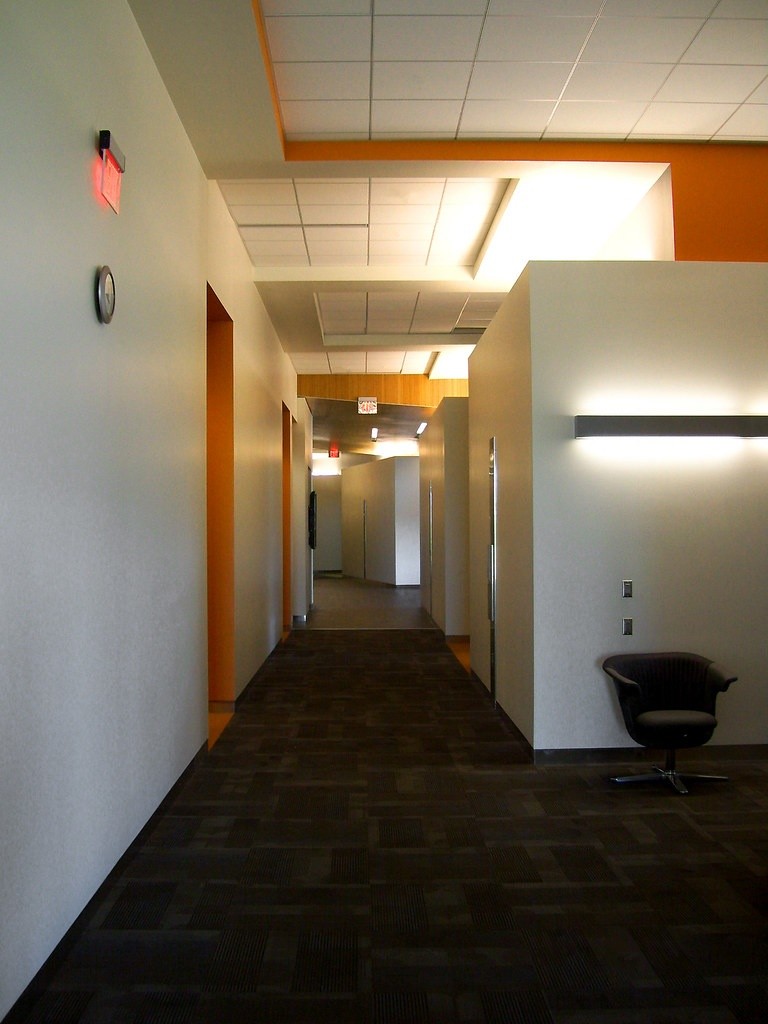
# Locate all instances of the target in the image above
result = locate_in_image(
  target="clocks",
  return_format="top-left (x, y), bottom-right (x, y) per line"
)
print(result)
top-left (96, 264), bottom-right (116, 324)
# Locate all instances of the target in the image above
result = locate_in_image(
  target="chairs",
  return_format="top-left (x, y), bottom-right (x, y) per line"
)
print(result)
top-left (603, 652), bottom-right (739, 794)
